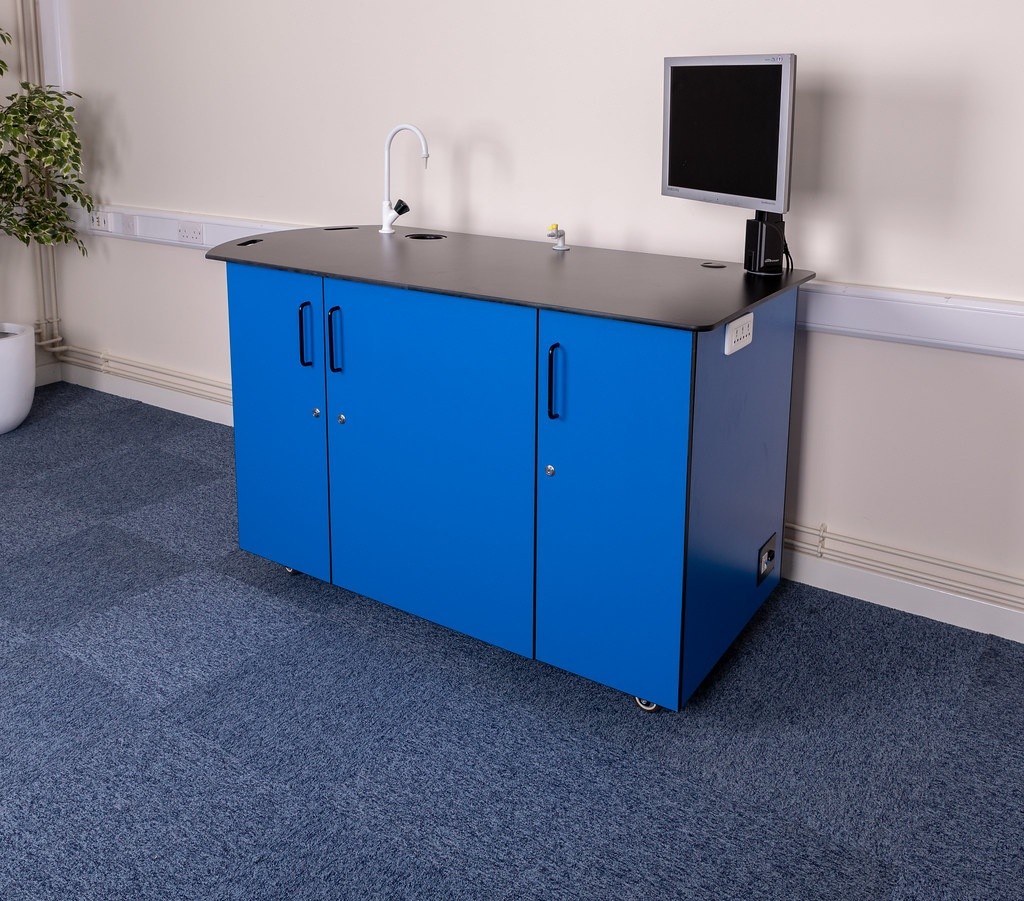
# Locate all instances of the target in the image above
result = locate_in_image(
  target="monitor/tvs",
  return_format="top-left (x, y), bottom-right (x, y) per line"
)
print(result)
top-left (661, 53), bottom-right (797, 213)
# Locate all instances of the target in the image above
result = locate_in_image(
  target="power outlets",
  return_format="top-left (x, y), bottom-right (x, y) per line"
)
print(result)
top-left (178, 221), bottom-right (203, 243)
top-left (90, 211), bottom-right (113, 231)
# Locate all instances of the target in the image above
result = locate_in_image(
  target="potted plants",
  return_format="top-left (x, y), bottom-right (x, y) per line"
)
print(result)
top-left (0, 30), bottom-right (98, 434)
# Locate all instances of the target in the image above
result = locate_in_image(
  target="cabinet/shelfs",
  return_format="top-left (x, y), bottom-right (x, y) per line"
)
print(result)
top-left (205, 223), bottom-right (816, 714)
